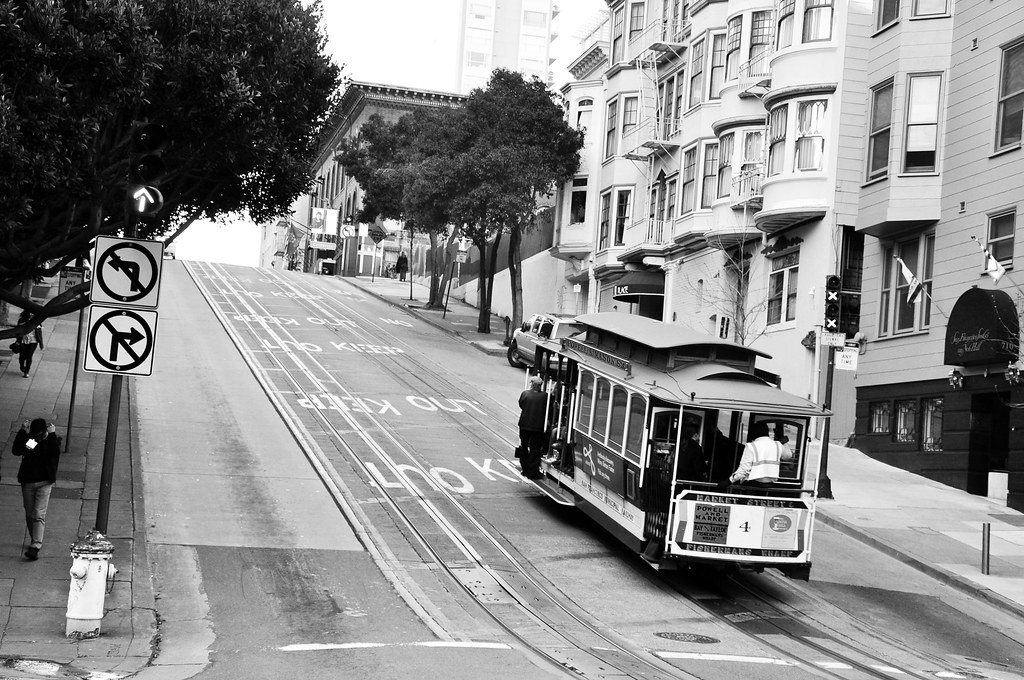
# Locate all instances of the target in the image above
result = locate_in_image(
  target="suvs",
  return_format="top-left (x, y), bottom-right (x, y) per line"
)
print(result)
top-left (504, 312), bottom-right (581, 375)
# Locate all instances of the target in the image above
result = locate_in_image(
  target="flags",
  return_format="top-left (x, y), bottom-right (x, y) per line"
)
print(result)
top-left (975, 238), bottom-right (1006, 286)
top-left (896, 257), bottom-right (923, 307)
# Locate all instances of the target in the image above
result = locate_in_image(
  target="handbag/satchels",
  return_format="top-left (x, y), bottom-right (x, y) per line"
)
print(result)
top-left (9, 342), bottom-right (20, 354)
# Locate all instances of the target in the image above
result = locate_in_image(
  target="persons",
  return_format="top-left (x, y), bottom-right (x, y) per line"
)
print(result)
top-left (12, 419), bottom-right (61, 559)
top-left (717, 421), bottom-right (793, 505)
top-left (667, 417), bottom-right (705, 502)
top-left (16, 309), bottom-right (44, 378)
top-left (396, 252), bottom-right (409, 282)
top-left (518, 376), bottom-right (569, 479)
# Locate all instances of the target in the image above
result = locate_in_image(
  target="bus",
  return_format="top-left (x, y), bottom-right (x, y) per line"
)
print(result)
top-left (513, 310), bottom-right (835, 582)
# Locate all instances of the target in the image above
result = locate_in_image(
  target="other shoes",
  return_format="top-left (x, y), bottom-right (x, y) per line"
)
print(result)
top-left (526, 472), bottom-right (543, 479)
top-left (20, 366), bottom-right (25, 372)
top-left (24, 373), bottom-right (29, 378)
top-left (24, 546), bottom-right (39, 561)
top-left (520, 470), bottom-right (527, 476)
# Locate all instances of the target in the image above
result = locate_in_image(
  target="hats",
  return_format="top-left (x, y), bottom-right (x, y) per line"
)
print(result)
top-left (530, 376), bottom-right (543, 385)
top-left (28, 418), bottom-right (47, 441)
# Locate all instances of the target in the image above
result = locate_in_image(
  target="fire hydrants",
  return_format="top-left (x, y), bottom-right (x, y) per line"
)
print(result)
top-left (64, 527), bottom-right (118, 641)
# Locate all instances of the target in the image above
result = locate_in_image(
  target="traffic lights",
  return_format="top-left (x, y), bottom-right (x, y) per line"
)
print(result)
top-left (825, 271), bottom-right (843, 335)
top-left (127, 117), bottom-right (171, 219)
